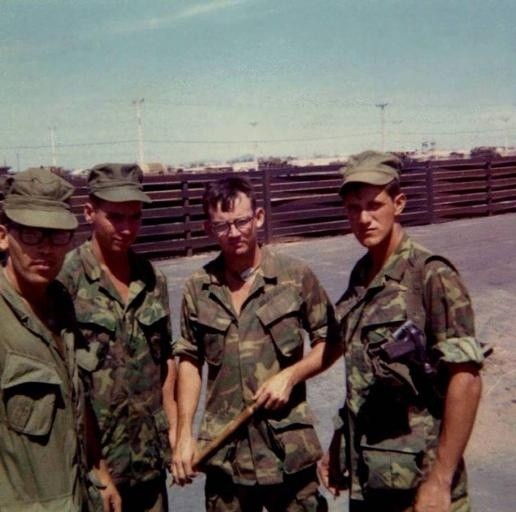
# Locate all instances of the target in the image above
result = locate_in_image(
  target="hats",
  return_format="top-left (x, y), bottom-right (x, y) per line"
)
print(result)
top-left (2, 166), bottom-right (80, 232)
top-left (86, 162), bottom-right (154, 206)
top-left (338, 150), bottom-right (405, 196)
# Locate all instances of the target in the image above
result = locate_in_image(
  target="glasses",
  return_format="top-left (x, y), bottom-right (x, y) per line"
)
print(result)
top-left (209, 213), bottom-right (255, 238)
top-left (16, 226), bottom-right (75, 248)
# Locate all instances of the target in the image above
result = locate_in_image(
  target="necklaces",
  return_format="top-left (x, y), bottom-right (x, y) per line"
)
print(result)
top-left (228, 261), bottom-right (258, 281)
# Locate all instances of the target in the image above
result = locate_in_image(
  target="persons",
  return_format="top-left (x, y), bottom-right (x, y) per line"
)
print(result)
top-left (53, 161), bottom-right (177, 512)
top-left (319, 150), bottom-right (484, 512)
top-left (168, 175), bottom-right (344, 512)
top-left (0, 167), bottom-right (122, 512)
